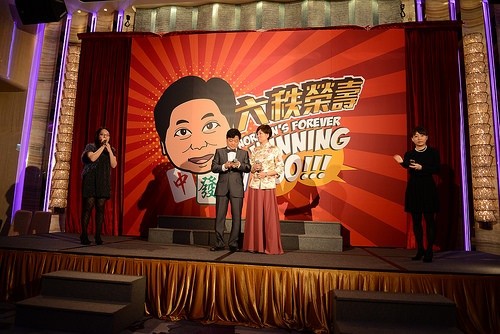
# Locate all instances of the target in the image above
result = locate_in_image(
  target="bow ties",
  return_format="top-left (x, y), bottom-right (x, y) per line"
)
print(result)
top-left (229, 149), bottom-right (236, 152)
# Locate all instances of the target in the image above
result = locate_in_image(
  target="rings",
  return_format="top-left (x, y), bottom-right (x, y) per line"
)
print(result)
top-left (259, 177), bottom-right (260, 179)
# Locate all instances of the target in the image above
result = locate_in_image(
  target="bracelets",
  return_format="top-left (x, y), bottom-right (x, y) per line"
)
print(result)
top-left (266, 172), bottom-right (268, 176)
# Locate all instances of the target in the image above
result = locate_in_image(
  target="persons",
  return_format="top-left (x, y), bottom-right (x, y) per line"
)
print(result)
top-left (242, 124), bottom-right (284, 255)
top-left (81, 128), bottom-right (117, 245)
top-left (394, 127), bottom-right (440, 263)
top-left (210, 129), bottom-right (252, 252)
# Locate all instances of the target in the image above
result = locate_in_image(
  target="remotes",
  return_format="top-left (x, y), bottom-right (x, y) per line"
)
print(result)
top-left (410, 159), bottom-right (415, 166)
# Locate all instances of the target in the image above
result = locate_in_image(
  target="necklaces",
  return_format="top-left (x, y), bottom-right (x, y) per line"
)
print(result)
top-left (415, 146), bottom-right (427, 152)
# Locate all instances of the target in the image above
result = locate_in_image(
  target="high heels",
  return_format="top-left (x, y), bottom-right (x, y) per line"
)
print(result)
top-left (412, 248), bottom-right (425, 260)
top-left (423, 248), bottom-right (433, 263)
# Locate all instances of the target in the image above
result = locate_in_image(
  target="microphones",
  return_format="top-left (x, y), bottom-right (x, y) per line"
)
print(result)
top-left (104, 140), bottom-right (106, 151)
top-left (256, 160), bottom-right (260, 173)
top-left (232, 159), bottom-right (237, 163)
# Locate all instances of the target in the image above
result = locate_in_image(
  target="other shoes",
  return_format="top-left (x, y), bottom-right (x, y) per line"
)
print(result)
top-left (81, 234), bottom-right (90, 245)
top-left (210, 245), bottom-right (225, 251)
top-left (95, 234), bottom-right (103, 245)
top-left (230, 247), bottom-right (239, 252)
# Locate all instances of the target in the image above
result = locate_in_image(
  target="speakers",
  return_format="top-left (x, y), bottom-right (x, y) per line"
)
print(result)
top-left (15, 0), bottom-right (68, 26)
